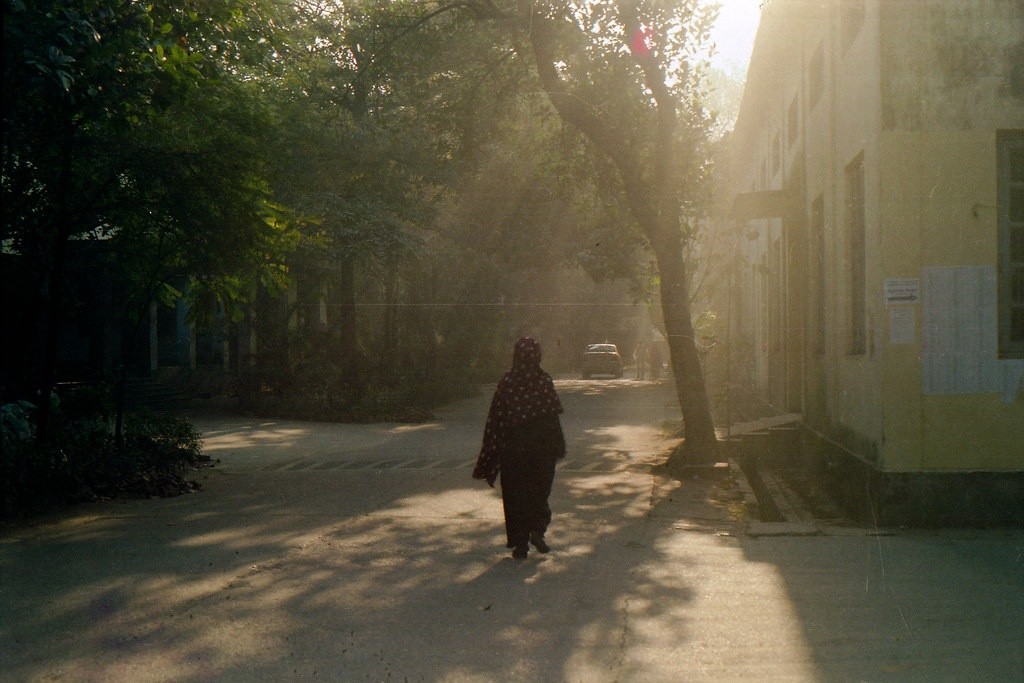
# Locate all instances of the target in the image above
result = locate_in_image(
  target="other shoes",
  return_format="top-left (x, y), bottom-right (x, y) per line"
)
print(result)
top-left (513, 547), bottom-right (527, 558)
top-left (530, 532), bottom-right (549, 553)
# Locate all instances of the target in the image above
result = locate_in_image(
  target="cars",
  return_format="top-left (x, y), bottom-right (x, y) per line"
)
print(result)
top-left (582, 343), bottom-right (624, 379)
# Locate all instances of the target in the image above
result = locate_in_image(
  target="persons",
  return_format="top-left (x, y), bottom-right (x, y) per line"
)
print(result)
top-left (472, 335), bottom-right (567, 558)
top-left (633, 341), bottom-right (663, 380)
